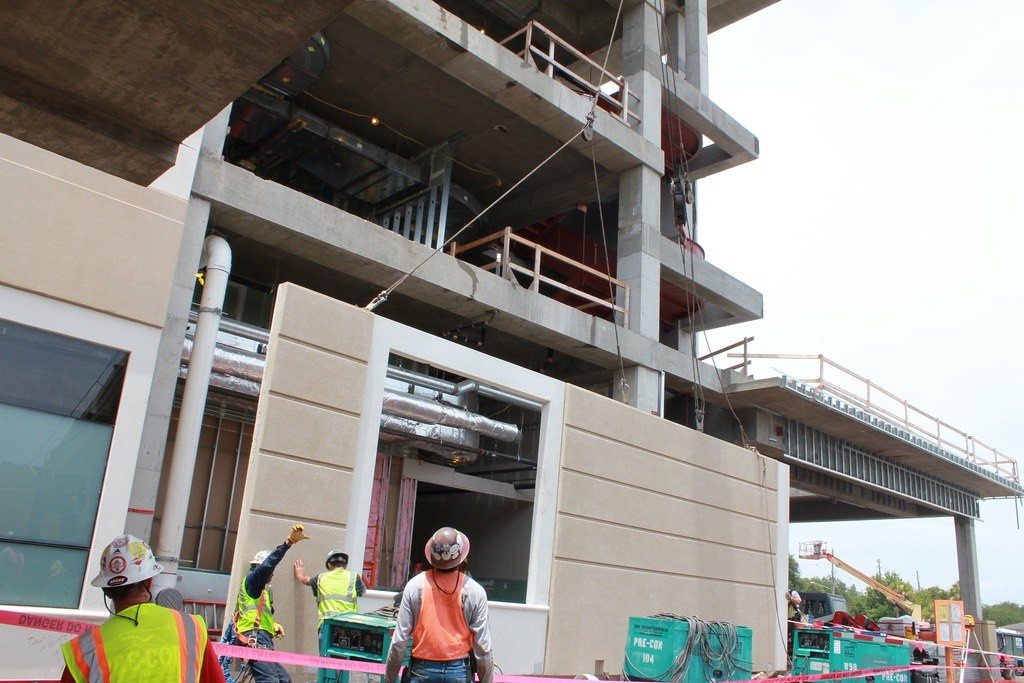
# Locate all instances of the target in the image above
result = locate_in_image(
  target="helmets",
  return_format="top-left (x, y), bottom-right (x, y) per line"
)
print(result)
top-left (250, 550), bottom-right (272, 564)
top-left (91, 534), bottom-right (164, 587)
top-left (326, 549), bottom-right (349, 571)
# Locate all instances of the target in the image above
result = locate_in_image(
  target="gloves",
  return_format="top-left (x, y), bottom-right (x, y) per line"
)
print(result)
top-left (274, 622), bottom-right (285, 639)
top-left (288, 524), bottom-right (311, 544)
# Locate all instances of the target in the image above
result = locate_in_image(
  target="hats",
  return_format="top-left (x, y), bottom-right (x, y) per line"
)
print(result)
top-left (425, 527), bottom-right (470, 569)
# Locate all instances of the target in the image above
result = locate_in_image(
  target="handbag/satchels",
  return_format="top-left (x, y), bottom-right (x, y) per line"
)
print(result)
top-left (793, 611), bottom-right (809, 624)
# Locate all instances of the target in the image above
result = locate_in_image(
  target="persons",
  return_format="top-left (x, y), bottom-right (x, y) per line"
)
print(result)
top-left (785, 589), bottom-right (801, 647)
top-left (60, 534), bottom-right (227, 683)
top-left (294, 547), bottom-right (366, 658)
top-left (232, 524), bottom-right (310, 683)
top-left (383, 527), bottom-right (495, 683)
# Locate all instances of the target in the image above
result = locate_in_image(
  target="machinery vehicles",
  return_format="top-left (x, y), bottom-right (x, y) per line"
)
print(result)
top-left (798, 540), bottom-right (932, 635)
top-left (788, 591), bottom-right (882, 658)
top-left (996, 627), bottom-right (1024, 680)
